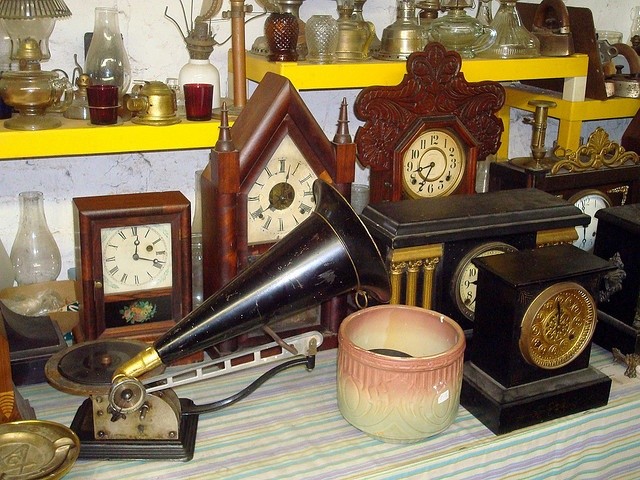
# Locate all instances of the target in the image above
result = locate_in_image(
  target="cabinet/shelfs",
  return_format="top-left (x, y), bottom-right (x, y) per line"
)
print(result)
top-left (2, 4), bottom-right (639, 157)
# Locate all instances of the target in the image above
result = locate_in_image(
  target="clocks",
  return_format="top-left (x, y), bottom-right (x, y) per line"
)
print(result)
top-left (199, 72), bottom-right (356, 368)
top-left (459, 244), bottom-right (617, 435)
top-left (353, 42), bottom-right (505, 203)
top-left (489, 101), bottom-right (639, 255)
top-left (359, 188), bottom-right (591, 344)
top-left (72, 190), bottom-right (205, 364)
